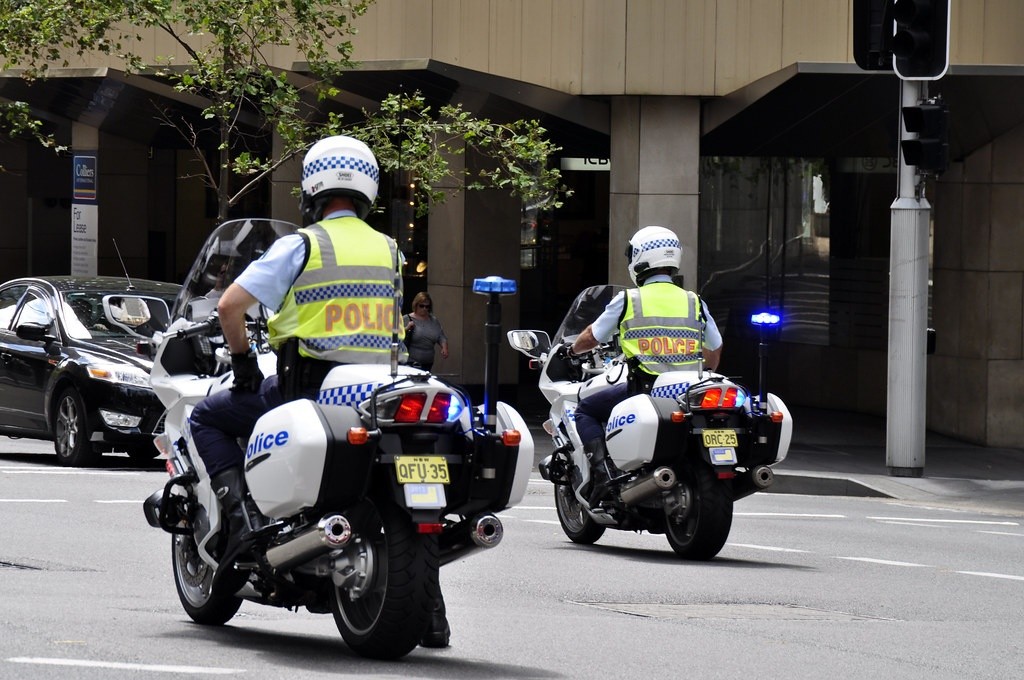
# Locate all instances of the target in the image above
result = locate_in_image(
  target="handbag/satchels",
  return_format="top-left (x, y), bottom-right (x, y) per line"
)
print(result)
top-left (404, 313), bottom-right (414, 349)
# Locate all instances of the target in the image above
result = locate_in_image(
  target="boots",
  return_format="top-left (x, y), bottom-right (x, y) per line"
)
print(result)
top-left (211, 467), bottom-right (273, 597)
top-left (583, 435), bottom-right (620, 509)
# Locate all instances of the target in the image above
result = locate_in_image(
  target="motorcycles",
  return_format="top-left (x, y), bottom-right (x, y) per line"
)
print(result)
top-left (102, 217), bottom-right (535, 661)
top-left (507, 284), bottom-right (794, 561)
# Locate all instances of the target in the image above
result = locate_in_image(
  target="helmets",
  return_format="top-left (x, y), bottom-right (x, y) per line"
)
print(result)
top-left (299, 134), bottom-right (380, 226)
top-left (628, 225), bottom-right (683, 288)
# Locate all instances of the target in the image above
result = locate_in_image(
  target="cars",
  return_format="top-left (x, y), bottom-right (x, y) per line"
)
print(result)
top-left (0, 276), bottom-right (185, 468)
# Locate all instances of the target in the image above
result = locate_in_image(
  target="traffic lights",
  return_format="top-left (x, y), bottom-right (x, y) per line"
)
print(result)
top-left (900, 139), bottom-right (942, 166)
top-left (900, 106), bottom-right (942, 133)
top-left (889, 0), bottom-right (950, 81)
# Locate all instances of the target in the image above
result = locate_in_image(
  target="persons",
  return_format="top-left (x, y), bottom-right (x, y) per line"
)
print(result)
top-left (191, 136), bottom-right (399, 596)
top-left (554, 225), bottom-right (726, 513)
top-left (401, 292), bottom-right (450, 372)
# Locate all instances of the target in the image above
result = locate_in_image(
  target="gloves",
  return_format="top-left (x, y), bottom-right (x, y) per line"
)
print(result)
top-left (556, 342), bottom-right (578, 361)
top-left (229, 347), bottom-right (264, 395)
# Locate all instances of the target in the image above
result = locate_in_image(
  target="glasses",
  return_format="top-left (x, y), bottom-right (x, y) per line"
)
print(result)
top-left (418, 303), bottom-right (431, 309)
